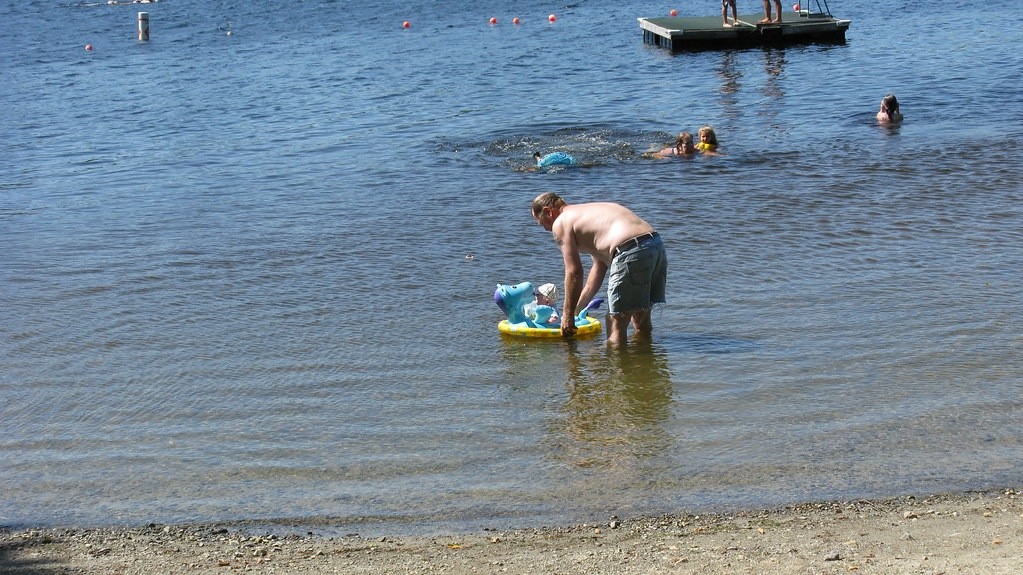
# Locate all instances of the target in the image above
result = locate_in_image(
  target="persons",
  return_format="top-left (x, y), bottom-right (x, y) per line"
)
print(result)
top-left (533, 283), bottom-right (561, 324)
top-left (531, 192), bottom-right (667, 348)
top-left (877, 94), bottom-right (904, 126)
top-left (721, 0), bottom-right (742, 28)
top-left (758, 0), bottom-right (783, 24)
top-left (652, 125), bottom-right (721, 159)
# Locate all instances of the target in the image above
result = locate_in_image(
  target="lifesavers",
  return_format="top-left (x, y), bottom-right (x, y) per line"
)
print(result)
top-left (537, 150), bottom-right (575, 167)
top-left (492, 281), bottom-right (606, 338)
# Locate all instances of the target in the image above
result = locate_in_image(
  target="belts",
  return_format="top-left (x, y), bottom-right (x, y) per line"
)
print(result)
top-left (613, 232), bottom-right (658, 257)
